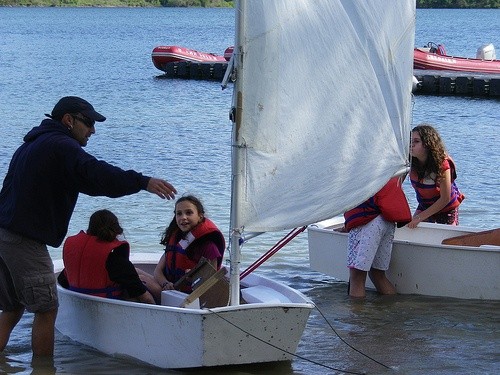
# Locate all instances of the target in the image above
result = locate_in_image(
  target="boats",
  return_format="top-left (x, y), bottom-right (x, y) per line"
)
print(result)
top-left (414, 41), bottom-right (500, 99)
top-left (151, 45), bottom-right (235, 79)
top-left (308, 211), bottom-right (500, 301)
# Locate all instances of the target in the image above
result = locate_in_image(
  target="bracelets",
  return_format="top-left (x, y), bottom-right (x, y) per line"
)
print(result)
top-left (414, 215), bottom-right (421, 221)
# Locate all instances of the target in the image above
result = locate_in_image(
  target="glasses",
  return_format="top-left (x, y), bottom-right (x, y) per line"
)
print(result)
top-left (71, 115), bottom-right (95, 128)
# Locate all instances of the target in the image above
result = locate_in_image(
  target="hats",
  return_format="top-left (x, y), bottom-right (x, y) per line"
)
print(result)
top-left (51, 96), bottom-right (106, 122)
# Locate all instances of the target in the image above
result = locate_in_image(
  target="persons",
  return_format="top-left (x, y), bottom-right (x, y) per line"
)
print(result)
top-left (135, 196), bottom-right (226, 303)
top-left (333, 196), bottom-right (396, 295)
top-left (406, 125), bottom-right (465, 228)
top-left (63, 209), bottom-right (153, 302)
top-left (0, 96), bottom-right (177, 375)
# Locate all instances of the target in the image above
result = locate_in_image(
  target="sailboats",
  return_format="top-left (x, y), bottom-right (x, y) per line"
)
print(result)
top-left (52, 0), bottom-right (422, 370)
